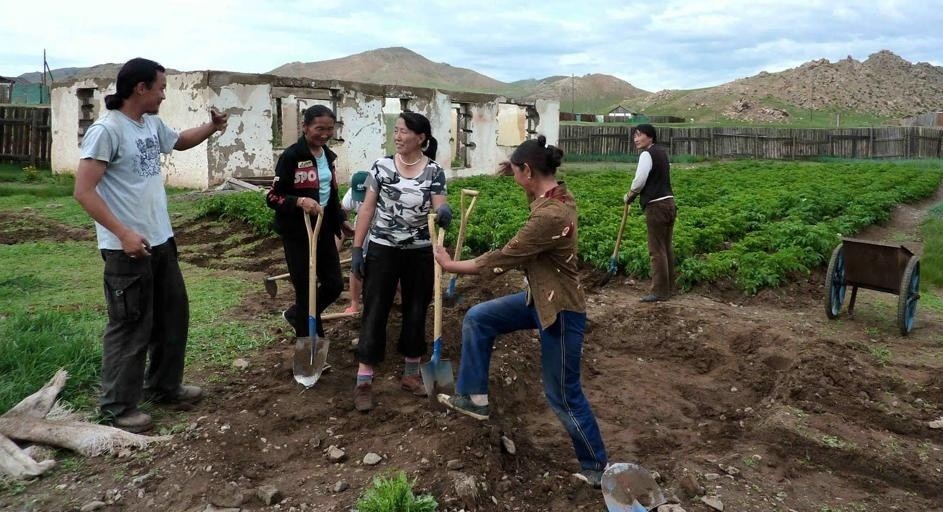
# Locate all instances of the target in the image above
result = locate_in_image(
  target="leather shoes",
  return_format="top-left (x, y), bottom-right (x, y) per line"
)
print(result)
top-left (168, 385), bottom-right (204, 403)
top-left (113, 409), bottom-right (154, 433)
top-left (639, 294), bottom-right (656, 303)
top-left (353, 383), bottom-right (374, 411)
top-left (401, 374), bottom-right (427, 396)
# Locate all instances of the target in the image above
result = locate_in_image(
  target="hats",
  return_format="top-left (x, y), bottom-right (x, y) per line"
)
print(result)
top-left (351, 171), bottom-right (369, 201)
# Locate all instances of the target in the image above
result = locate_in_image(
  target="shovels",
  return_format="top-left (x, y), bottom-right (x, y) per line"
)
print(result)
top-left (420, 214), bottom-right (455, 413)
top-left (600, 463), bottom-right (668, 512)
top-left (447, 189), bottom-right (480, 297)
top-left (600, 203), bottom-right (631, 287)
top-left (293, 205), bottom-right (330, 389)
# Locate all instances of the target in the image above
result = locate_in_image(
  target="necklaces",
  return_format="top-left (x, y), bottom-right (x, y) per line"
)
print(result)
top-left (398, 153), bottom-right (423, 167)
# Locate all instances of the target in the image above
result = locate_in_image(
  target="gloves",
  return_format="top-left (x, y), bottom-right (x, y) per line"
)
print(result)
top-left (623, 192), bottom-right (632, 204)
top-left (351, 247), bottom-right (367, 282)
top-left (434, 204), bottom-right (453, 230)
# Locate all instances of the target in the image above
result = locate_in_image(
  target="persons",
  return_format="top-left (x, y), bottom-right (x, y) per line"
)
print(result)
top-left (623, 123), bottom-right (678, 300)
top-left (73, 57), bottom-right (229, 432)
top-left (334, 170), bottom-right (402, 315)
top-left (432, 133), bottom-right (608, 487)
top-left (349, 107), bottom-right (453, 413)
top-left (265, 105), bottom-right (345, 373)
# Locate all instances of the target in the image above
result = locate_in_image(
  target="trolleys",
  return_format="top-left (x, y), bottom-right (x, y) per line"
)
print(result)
top-left (824, 236), bottom-right (922, 336)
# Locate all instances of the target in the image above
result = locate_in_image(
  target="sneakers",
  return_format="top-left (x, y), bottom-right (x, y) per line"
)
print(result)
top-left (282, 304), bottom-right (298, 333)
top-left (571, 462), bottom-right (609, 487)
top-left (322, 362), bottom-right (332, 373)
top-left (345, 306), bottom-right (358, 313)
top-left (437, 393), bottom-right (490, 420)
